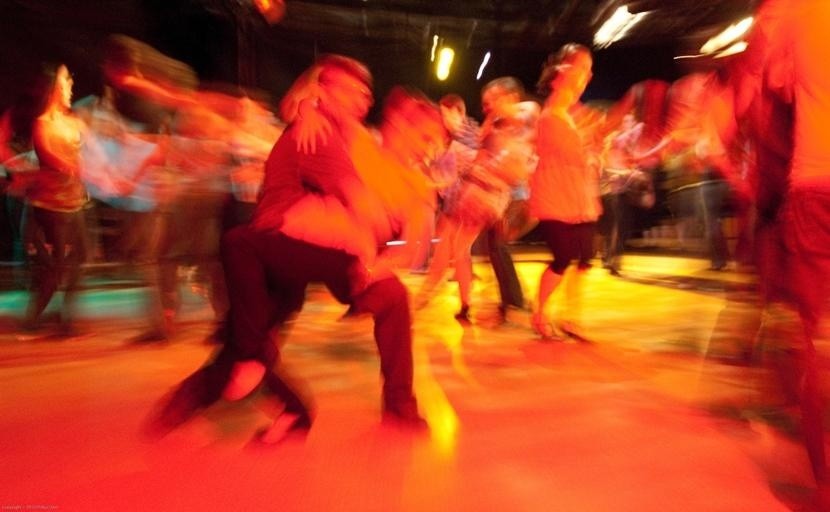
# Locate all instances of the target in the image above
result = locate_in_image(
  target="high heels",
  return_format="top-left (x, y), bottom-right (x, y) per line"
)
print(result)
top-left (455, 304), bottom-right (470, 319)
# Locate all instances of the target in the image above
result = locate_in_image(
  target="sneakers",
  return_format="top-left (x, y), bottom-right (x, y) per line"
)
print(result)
top-left (532, 313), bottom-right (592, 343)
